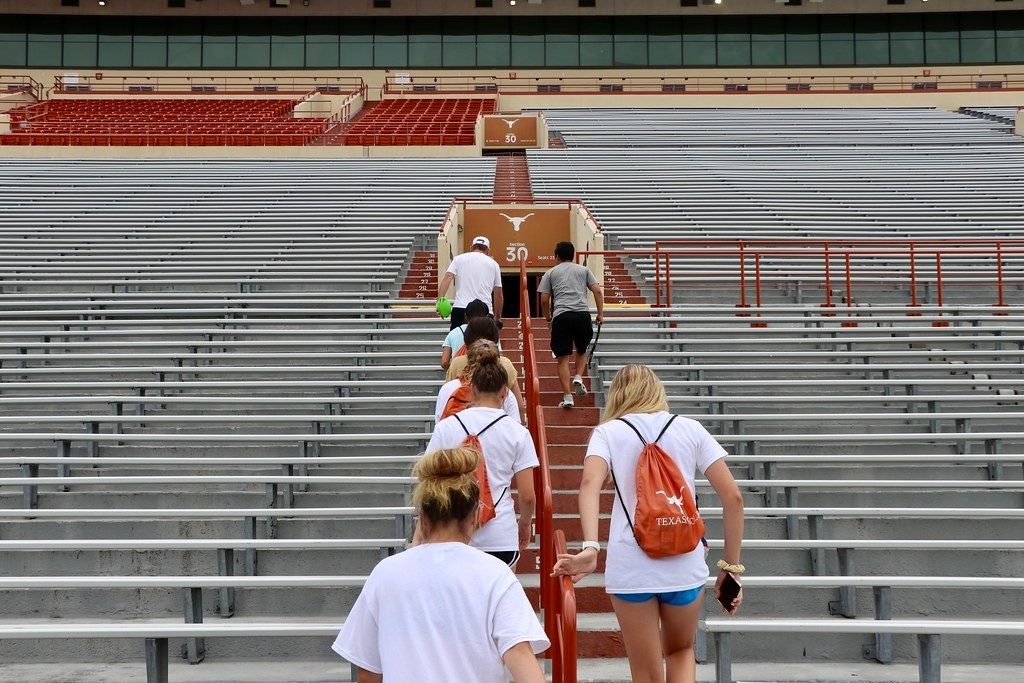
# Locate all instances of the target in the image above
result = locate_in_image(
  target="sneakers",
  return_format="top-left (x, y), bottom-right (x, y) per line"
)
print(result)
top-left (559, 395), bottom-right (574, 408)
top-left (572, 375), bottom-right (587, 399)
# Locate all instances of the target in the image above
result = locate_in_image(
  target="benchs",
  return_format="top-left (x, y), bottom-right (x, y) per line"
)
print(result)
top-left (0, 156), bottom-right (497, 682)
top-left (518, 103), bottom-right (1024, 683)
top-left (0, 99), bottom-right (498, 146)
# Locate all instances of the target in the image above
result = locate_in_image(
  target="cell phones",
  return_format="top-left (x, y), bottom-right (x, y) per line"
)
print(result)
top-left (717, 572), bottom-right (742, 613)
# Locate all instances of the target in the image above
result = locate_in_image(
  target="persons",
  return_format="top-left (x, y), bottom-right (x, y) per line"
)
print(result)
top-left (330, 446), bottom-right (552, 683)
top-left (537, 241), bottom-right (603, 408)
top-left (440, 297), bottom-right (503, 371)
top-left (411, 350), bottom-right (540, 574)
top-left (435, 235), bottom-right (503, 332)
top-left (436, 340), bottom-right (523, 424)
top-left (445, 317), bottom-right (526, 425)
top-left (550, 363), bottom-right (744, 683)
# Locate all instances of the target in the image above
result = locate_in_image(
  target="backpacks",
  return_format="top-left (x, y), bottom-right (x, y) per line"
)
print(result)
top-left (609, 413), bottom-right (706, 558)
top-left (452, 326), bottom-right (468, 359)
top-left (440, 374), bottom-right (477, 421)
top-left (453, 412), bottom-right (509, 531)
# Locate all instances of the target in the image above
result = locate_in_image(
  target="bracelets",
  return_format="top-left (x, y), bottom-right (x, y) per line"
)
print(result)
top-left (546, 318), bottom-right (553, 323)
top-left (717, 559), bottom-right (745, 573)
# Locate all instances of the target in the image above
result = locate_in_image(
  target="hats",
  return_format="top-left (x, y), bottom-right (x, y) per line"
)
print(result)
top-left (472, 236), bottom-right (489, 249)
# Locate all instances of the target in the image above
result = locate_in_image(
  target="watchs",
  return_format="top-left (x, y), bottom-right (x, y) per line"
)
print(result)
top-left (582, 541), bottom-right (600, 552)
